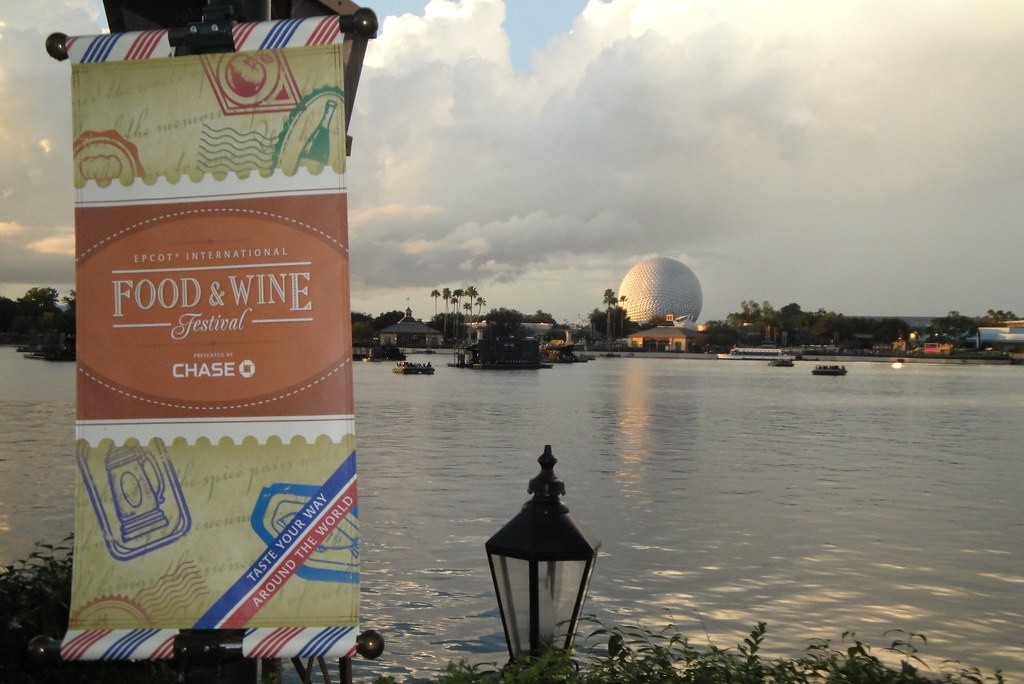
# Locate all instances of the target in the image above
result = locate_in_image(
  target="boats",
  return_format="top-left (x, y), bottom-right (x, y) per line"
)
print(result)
top-left (812, 364), bottom-right (848, 376)
top-left (767, 357), bottom-right (794, 367)
top-left (716, 347), bottom-right (793, 360)
top-left (392, 320), bottom-right (596, 374)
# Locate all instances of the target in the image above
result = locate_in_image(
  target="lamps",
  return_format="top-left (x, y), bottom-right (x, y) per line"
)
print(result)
top-left (486, 444), bottom-right (601, 683)
